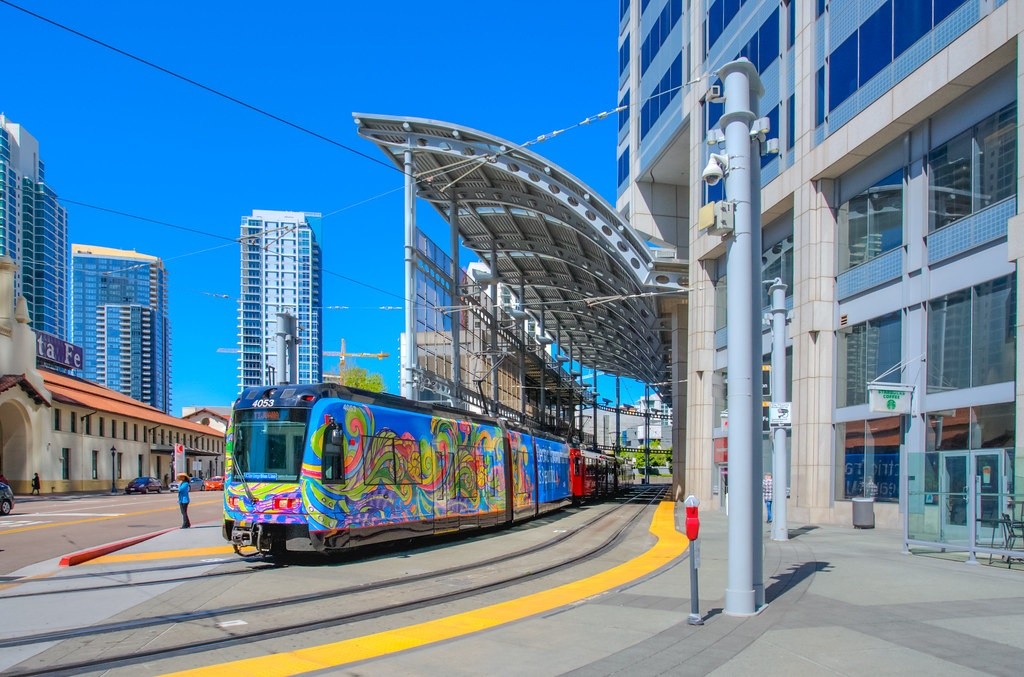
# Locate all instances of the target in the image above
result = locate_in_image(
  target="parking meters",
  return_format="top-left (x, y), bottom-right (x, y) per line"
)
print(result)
top-left (684, 493), bottom-right (704, 626)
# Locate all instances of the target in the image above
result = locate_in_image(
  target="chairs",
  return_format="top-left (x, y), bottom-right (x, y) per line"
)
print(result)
top-left (1004, 514), bottom-right (1024, 569)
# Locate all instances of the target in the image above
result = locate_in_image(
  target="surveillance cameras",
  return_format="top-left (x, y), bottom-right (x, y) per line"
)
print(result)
top-left (707, 86), bottom-right (720, 102)
top-left (702, 164), bottom-right (723, 186)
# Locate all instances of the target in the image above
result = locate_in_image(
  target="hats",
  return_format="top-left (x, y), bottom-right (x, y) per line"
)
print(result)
top-left (177, 472), bottom-right (190, 481)
top-left (34, 473), bottom-right (38, 475)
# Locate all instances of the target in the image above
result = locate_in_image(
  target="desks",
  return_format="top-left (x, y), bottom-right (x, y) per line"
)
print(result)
top-left (975, 518), bottom-right (1024, 563)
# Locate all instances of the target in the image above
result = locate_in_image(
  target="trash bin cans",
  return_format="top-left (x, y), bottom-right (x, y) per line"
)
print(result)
top-left (851, 497), bottom-right (875, 529)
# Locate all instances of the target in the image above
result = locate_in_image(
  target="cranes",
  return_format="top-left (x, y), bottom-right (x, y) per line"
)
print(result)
top-left (322, 338), bottom-right (390, 385)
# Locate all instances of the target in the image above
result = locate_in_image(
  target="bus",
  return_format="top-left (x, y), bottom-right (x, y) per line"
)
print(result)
top-left (221, 381), bottom-right (572, 561)
top-left (570, 449), bottom-right (633, 504)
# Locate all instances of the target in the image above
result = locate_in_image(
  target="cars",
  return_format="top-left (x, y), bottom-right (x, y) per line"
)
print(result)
top-left (168, 476), bottom-right (205, 493)
top-left (0, 482), bottom-right (16, 516)
top-left (126, 476), bottom-right (162, 493)
top-left (204, 476), bottom-right (224, 491)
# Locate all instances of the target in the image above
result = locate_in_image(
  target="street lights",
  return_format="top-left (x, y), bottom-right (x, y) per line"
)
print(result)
top-left (110, 445), bottom-right (118, 493)
top-left (215, 457), bottom-right (218, 476)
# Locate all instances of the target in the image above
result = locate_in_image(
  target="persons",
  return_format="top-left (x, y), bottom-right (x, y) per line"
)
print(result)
top-left (164, 474), bottom-right (169, 487)
top-left (762, 473), bottom-right (773, 523)
top-left (30, 473), bottom-right (40, 495)
top-left (177, 472), bottom-right (191, 528)
top-left (0, 475), bottom-right (6, 482)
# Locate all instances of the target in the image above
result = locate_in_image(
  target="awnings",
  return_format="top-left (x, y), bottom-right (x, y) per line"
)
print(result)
top-left (150, 444), bottom-right (222, 476)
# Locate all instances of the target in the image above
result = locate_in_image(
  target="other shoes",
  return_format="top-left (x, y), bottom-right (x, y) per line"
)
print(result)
top-left (180, 525), bottom-right (191, 529)
top-left (36, 493), bottom-right (40, 496)
top-left (30, 493), bottom-right (33, 495)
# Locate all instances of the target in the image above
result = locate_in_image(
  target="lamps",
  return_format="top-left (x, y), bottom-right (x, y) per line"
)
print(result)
top-left (498, 308), bottom-right (531, 330)
top-left (563, 369), bottom-right (581, 383)
top-left (598, 398), bottom-right (613, 407)
top-left (621, 404), bottom-right (634, 412)
top-left (574, 383), bottom-right (592, 393)
top-left (549, 354), bottom-right (570, 369)
top-left (651, 408), bottom-right (663, 414)
top-left (526, 334), bottom-right (553, 353)
top-left (586, 392), bottom-right (601, 400)
top-left (458, 269), bottom-right (497, 299)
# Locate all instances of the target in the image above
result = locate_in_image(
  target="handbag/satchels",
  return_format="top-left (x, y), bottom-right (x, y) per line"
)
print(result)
top-left (32, 483), bottom-right (35, 487)
